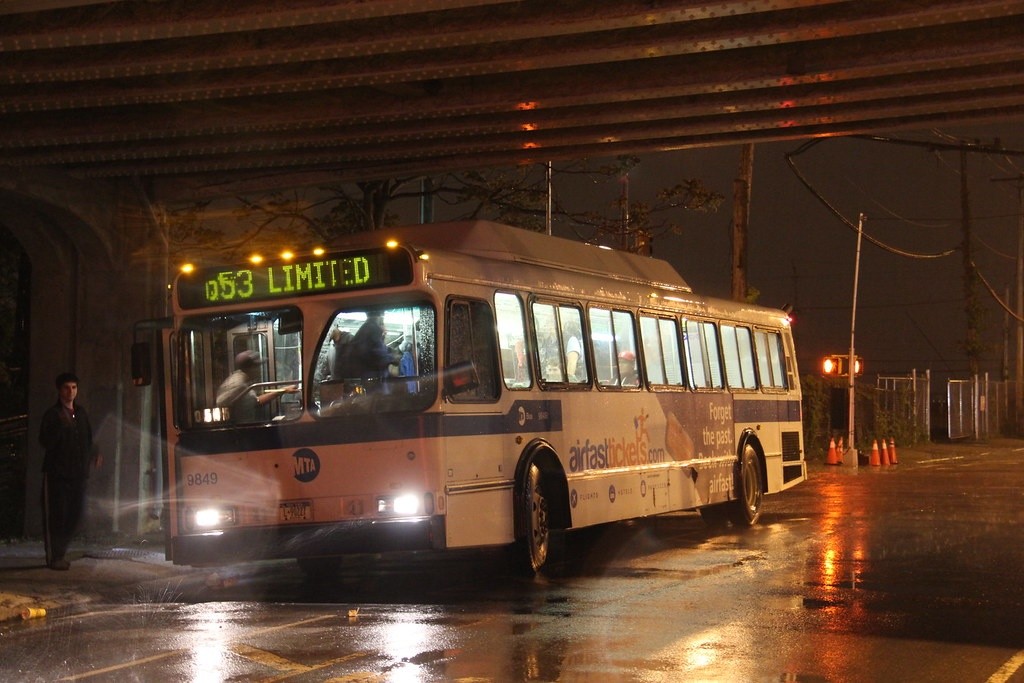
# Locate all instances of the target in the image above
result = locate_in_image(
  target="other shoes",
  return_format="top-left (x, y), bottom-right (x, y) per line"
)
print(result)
top-left (48, 556), bottom-right (70, 570)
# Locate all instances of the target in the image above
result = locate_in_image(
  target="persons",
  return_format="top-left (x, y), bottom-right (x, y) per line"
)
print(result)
top-left (40, 371), bottom-right (102, 571)
top-left (329, 308), bottom-right (420, 399)
top-left (216, 351), bottom-right (302, 424)
top-left (512, 314), bottom-right (588, 383)
top-left (608, 350), bottom-right (638, 385)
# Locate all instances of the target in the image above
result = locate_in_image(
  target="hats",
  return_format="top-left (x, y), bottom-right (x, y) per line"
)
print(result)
top-left (55, 372), bottom-right (81, 386)
top-left (235, 349), bottom-right (267, 368)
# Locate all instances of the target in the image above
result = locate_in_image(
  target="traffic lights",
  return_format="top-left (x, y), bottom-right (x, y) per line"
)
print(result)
top-left (820, 354), bottom-right (863, 378)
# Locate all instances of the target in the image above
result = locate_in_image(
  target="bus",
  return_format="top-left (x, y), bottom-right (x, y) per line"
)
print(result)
top-left (131, 220), bottom-right (808, 577)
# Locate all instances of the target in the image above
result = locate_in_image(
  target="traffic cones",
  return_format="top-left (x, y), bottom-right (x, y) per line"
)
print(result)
top-left (888, 437), bottom-right (898, 464)
top-left (870, 439), bottom-right (881, 465)
top-left (824, 437), bottom-right (844, 465)
top-left (881, 438), bottom-right (890, 464)
